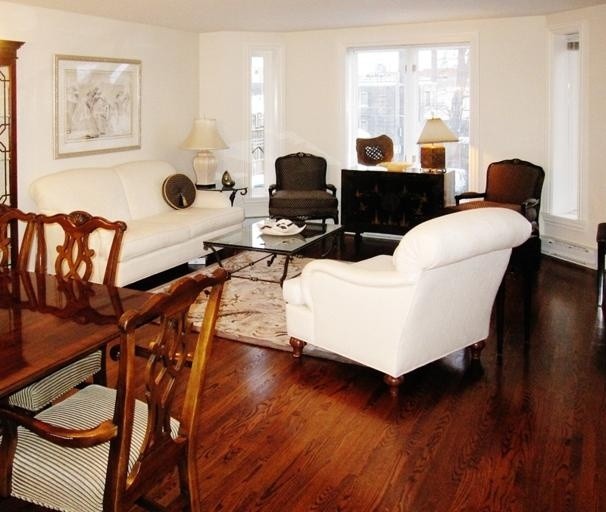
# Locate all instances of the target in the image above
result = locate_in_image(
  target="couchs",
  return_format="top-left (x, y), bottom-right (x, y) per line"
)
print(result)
top-left (281, 208), bottom-right (534, 399)
top-left (26, 158), bottom-right (246, 291)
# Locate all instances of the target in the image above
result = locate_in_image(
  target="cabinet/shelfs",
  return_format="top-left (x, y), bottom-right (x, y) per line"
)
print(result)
top-left (341, 164), bottom-right (455, 243)
top-left (2, 40), bottom-right (25, 271)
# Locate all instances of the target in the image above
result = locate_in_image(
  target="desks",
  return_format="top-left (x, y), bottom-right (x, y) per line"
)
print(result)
top-left (206, 183), bottom-right (248, 207)
top-left (1, 264), bottom-right (162, 404)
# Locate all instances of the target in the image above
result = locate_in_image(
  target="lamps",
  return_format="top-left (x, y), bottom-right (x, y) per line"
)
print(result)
top-left (418, 115), bottom-right (461, 173)
top-left (180, 115), bottom-right (230, 189)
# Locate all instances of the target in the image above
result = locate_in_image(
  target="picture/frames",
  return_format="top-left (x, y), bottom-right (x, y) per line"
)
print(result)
top-left (52, 52), bottom-right (144, 161)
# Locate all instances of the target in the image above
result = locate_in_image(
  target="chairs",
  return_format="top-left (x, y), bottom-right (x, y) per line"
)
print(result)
top-left (454, 156), bottom-right (545, 243)
top-left (266, 152), bottom-right (340, 225)
top-left (0, 204), bottom-right (39, 267)
top-left (5, 208), bottom-right (128, 416)
top-left (0, 268), bottom-right (227, 510)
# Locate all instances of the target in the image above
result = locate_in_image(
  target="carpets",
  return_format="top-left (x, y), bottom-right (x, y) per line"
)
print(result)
top-left (140, 250), bottom-right (363, 366)
